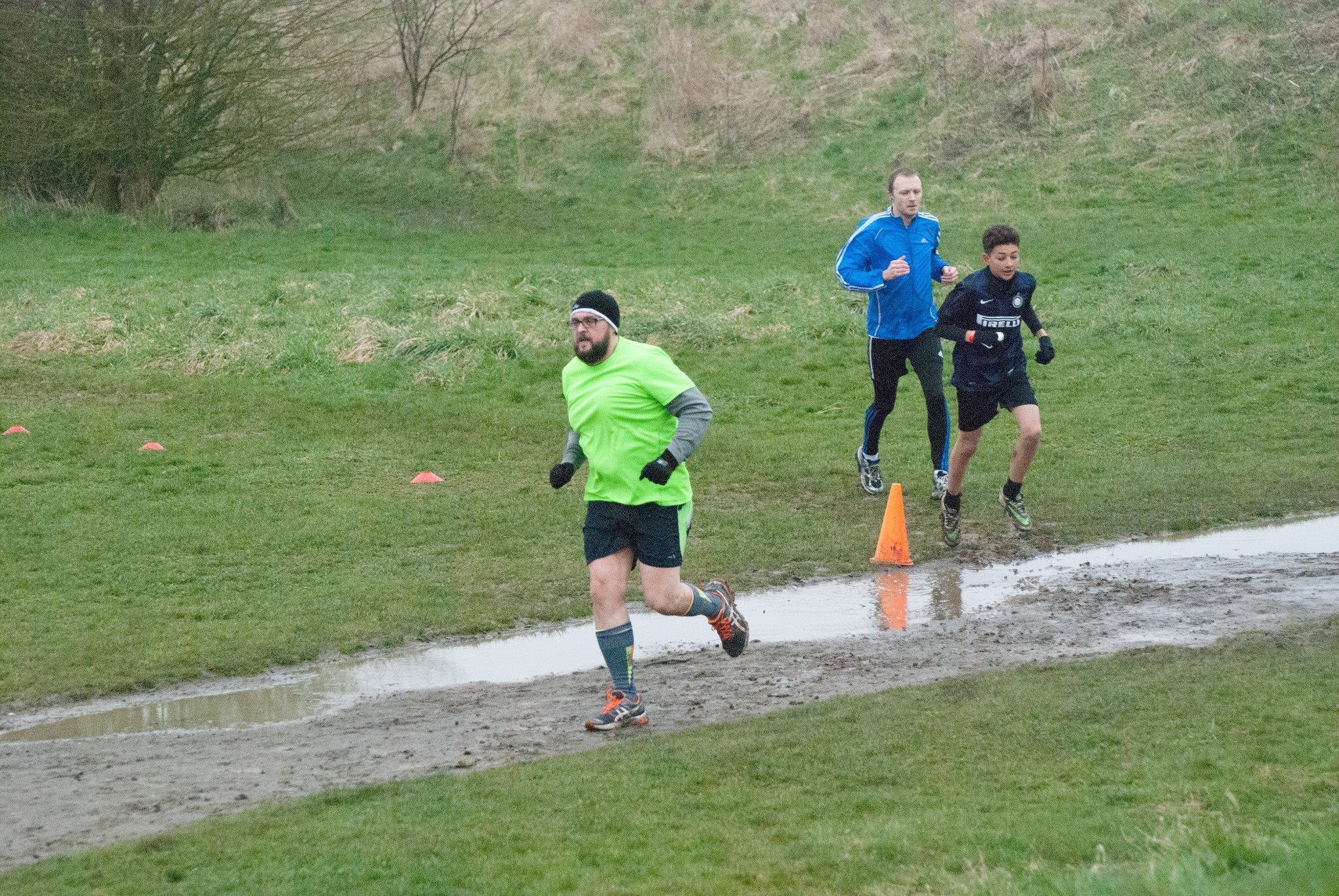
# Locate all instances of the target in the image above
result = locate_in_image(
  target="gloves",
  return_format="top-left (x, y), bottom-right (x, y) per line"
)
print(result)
top-left (973, 331), bottom-right (998, 352)
top-left (1035, 336), bottom-right (1055, 364)
top-left (550, 463), bottom-right (574, 489)
top-left (638, 449), bottom-right (679, 485)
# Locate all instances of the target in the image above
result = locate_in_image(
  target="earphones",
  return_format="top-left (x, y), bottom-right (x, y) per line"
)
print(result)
top-left (890, 195), bottom-right (893, 200)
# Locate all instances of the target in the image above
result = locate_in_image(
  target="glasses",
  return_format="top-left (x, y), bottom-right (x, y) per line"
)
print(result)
top-left (567, 317), bottom-right (603, 330)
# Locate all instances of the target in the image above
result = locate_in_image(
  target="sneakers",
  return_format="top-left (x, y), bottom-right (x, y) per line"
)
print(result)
top-left (586, 685), bottom-right (649, 730)
top-left (705, 579), bottom-right (749, 658)
top-left (856, 445), bottom-right (884, 493)
top-left (998, 485), bottom-right (1033, 531)
top-left (931, 469), bottom-right (948, 500)
top-left (941, 491), bottom-right (961, 548)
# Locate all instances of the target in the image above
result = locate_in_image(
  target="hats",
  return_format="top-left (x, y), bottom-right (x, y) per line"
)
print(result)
top-left (570, 289), bottom-right (620, 334)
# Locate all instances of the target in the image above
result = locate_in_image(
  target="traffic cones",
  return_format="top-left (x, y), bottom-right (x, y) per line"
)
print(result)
top-left (871, 482), bottom-right (914, 566)
top-left (875, 571), bottom-right (910, 630)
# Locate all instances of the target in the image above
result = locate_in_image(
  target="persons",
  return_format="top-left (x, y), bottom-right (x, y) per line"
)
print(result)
top-left (550, 291), bottom-right (749, 731)
top-left (834, 168), bottom-right (958, 501)
top-left (936, 224), bottom-right (1056, 548)
